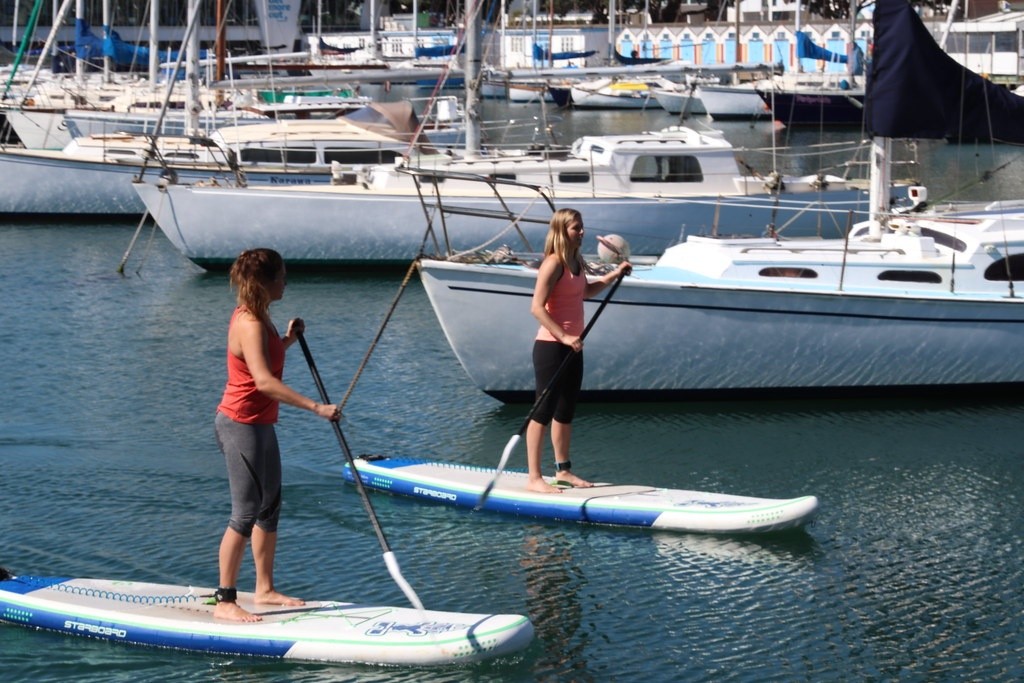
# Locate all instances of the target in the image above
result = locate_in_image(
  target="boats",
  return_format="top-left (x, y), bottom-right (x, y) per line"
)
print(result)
top-left (340, 452), bottom-right (820, 538)
top-left (0, 572), bottom-right (536, 672)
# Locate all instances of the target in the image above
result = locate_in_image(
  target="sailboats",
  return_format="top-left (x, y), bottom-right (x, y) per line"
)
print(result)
top-left (0, 0), bottom-right (1021, 219)
top-left (410, 0), bottom-right (1024, 406)
top-left (130, 1), bottom-right (928, 275)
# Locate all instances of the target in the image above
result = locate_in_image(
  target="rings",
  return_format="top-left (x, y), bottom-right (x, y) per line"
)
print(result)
top-left (335, 412), bottom-right (338, 416)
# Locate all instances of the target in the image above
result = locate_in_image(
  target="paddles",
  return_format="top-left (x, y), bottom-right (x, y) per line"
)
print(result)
top-left (472, 265), bottom-right (637, 512)
top-left (298, 329), bottom-right (426, 612)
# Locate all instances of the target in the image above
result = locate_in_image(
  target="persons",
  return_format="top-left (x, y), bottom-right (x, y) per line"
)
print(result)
top-left (527, 209), bottom-right (632, 493)
top-left (850, 39), bottom-right (874, 76)
top-left (214, 248), bottom-right (342, 622)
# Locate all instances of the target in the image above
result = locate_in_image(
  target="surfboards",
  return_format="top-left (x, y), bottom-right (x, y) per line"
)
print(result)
top-left (0, 570), bottom-right (533, 668)
top-left (341, 457), bottom-right (816, 537)
